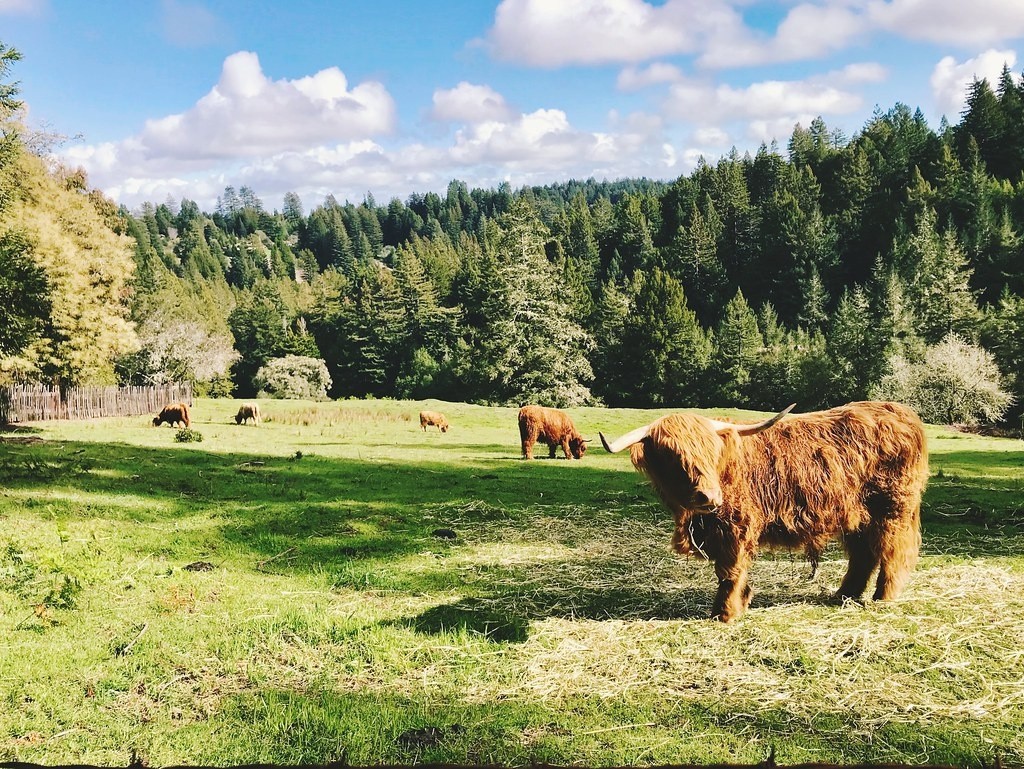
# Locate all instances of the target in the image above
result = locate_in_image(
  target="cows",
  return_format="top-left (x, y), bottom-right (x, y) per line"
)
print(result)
top-left (230, 402), bottom-right (263, 427)
top-left (517, 406), bottom-right (593, 461)
top-left (152, 403), bottom-right (190, 428)
top-left (420, 410), bottom-right (449, 433)
top-left (599, 401), bottom-right (931, 623)
